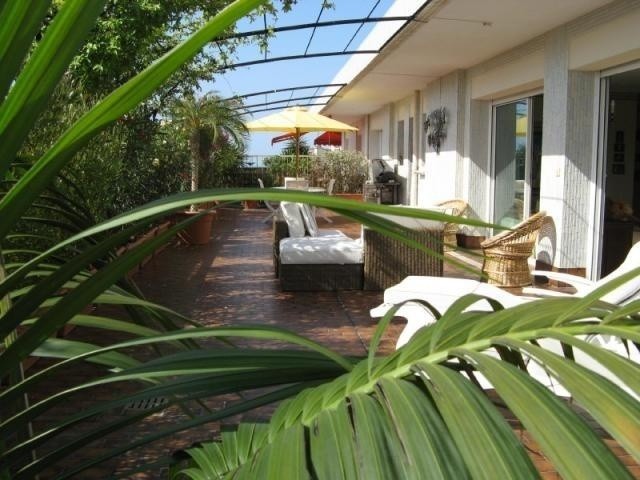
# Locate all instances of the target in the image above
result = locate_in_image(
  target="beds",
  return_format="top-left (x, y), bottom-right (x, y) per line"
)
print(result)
top-left (271, 215), bottom-right (365, 291)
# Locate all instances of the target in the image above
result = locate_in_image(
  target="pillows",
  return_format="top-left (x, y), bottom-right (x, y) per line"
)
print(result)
top-left (365, 203), bottom-right (447, 232)
top-left (280, 200), bottom-right (319, 237)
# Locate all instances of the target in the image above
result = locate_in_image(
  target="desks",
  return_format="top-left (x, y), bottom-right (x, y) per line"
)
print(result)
top-left (362, 182), bottom-right (401, 205)
top-left (269, 186), bottom-right (326, 193)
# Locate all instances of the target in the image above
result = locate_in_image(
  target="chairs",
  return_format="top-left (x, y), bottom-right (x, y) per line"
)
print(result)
top-left (363, 226), bottom-right (445, 291)
top-left (310, 178), bottom-right (336, 223)
top-left (435, 199), bottom-right (469, 252)
top-left (368, 240), bottom-right (640, 406)
top-left (480, 210), bottom-right (547, 288)
top-left (257, 177), bottom-right (285, 224)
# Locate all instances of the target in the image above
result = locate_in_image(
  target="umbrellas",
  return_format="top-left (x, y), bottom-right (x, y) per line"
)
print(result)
top-left (313, 130), bottom-right (342, 147)
top-left (233, 103), bottom-right (362, 178)
